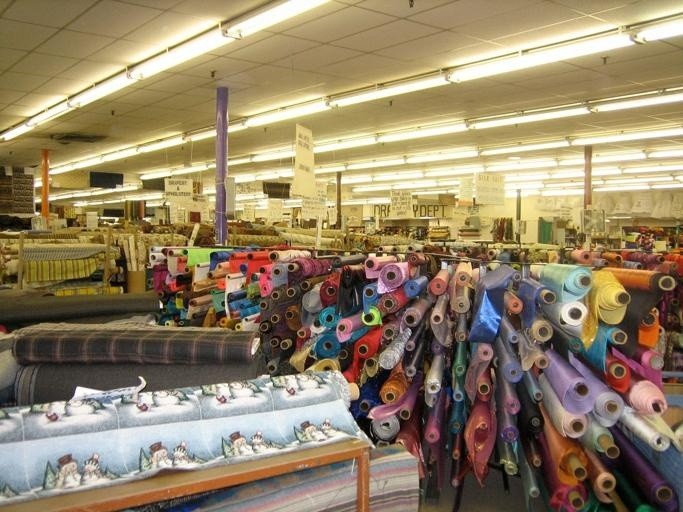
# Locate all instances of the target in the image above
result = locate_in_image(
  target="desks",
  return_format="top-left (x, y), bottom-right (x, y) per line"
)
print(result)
top-left (0, 439), bottom-right (370, 512)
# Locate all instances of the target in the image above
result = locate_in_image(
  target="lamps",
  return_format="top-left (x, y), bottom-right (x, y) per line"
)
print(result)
top-left (0, 0), bottom-right (683, 195)
